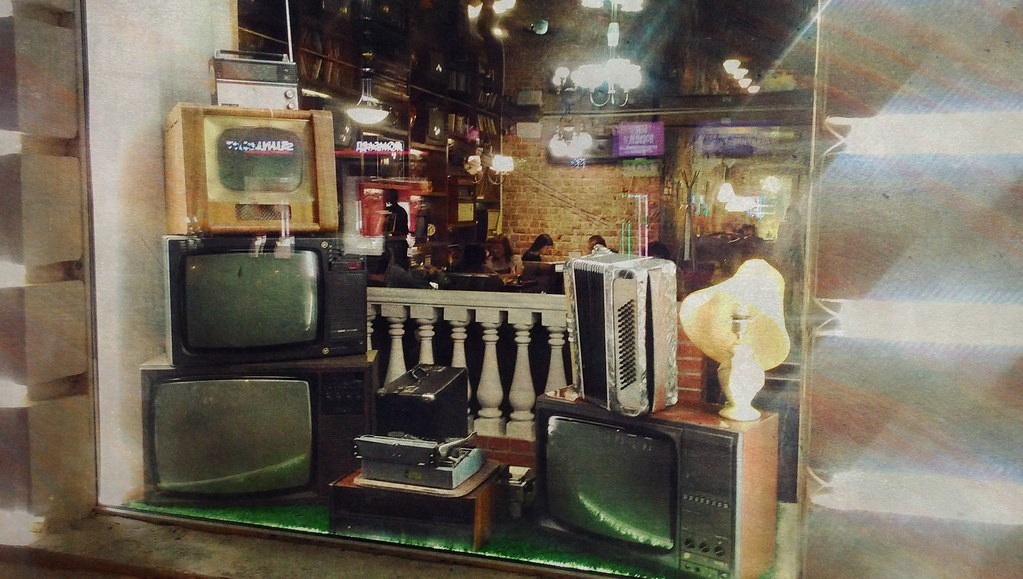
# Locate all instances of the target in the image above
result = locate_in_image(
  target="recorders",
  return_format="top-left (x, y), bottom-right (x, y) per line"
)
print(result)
top-left (208, 0), bottom-right (299, 110)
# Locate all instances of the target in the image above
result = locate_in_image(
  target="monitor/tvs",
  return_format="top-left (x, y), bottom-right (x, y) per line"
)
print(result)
top-left (163, 233), bottom-right (367, 368)
top-left (447, 271), bottom-right (504, 292)
top-left (535, 383), bottom-right (778, 579)
top-left (522, 261), bottom-right (566, 295)
top-left (140, 350), bottom-right (380, 509)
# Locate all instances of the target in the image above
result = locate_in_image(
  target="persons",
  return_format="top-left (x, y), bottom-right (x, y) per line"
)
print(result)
top-left (485, 234), bottom-right (523, 279)
top-left (642, 240), bottom-right (685, 302)
top-left (382, 189), bottom-right (410, 275)
top-left (449, 241), bottom-right (493, 273)
top-left (522, 234), bottom-right (553, 262)
top-left (587, 235), bottom-right (619, 254)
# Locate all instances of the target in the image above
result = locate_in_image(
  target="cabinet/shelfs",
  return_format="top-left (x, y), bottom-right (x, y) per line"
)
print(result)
top-left (237, 23), bottom-right (504, 239)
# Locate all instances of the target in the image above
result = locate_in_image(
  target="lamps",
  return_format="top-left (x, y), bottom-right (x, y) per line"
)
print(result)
top-left (463, 154), bottom-right (515, 186)
top-left (552, 67), bottom-right (570, 96)
top-left (467, 1), bottom-right (516, 45)
top-left (345, 42), bottom-right (391, 126)
top-left (680, 256), bottom-right (793, 424)
top-left (548, 119), bottom-right (595, 169)
top-left (570, 0), bottom-right (644, 108)
top-left (722, 53), bottom-right (762, 95)
